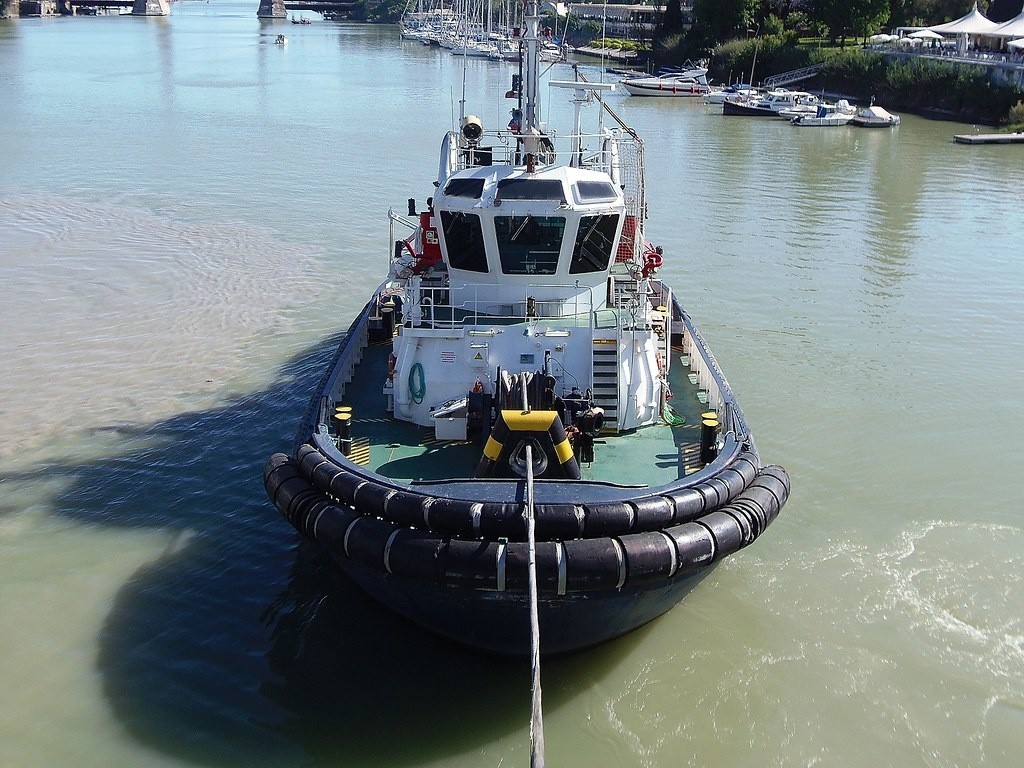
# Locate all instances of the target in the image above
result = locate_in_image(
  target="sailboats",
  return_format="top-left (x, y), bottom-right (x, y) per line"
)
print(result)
top-left (397, 0), bottom-right (572, 66)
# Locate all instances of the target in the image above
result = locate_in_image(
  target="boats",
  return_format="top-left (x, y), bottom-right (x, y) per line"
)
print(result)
top-left (606, 55), bottom-right (902, 128)
top-left (261, 1), bottom-right (794, 658)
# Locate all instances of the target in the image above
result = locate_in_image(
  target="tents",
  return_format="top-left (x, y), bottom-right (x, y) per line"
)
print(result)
top-left (920, 1), bottom-right (1024, 53)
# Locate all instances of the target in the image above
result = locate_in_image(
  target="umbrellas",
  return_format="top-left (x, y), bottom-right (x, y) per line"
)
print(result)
top-left (869, 28), bottom-right (945, 47)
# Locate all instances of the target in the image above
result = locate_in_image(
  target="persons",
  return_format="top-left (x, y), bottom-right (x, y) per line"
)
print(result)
top-left (544, 25), bottom-right (553, 41)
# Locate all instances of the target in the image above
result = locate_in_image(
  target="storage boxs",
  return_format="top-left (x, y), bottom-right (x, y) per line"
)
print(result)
top-left (428, 394), bottom-right (470, 440)
